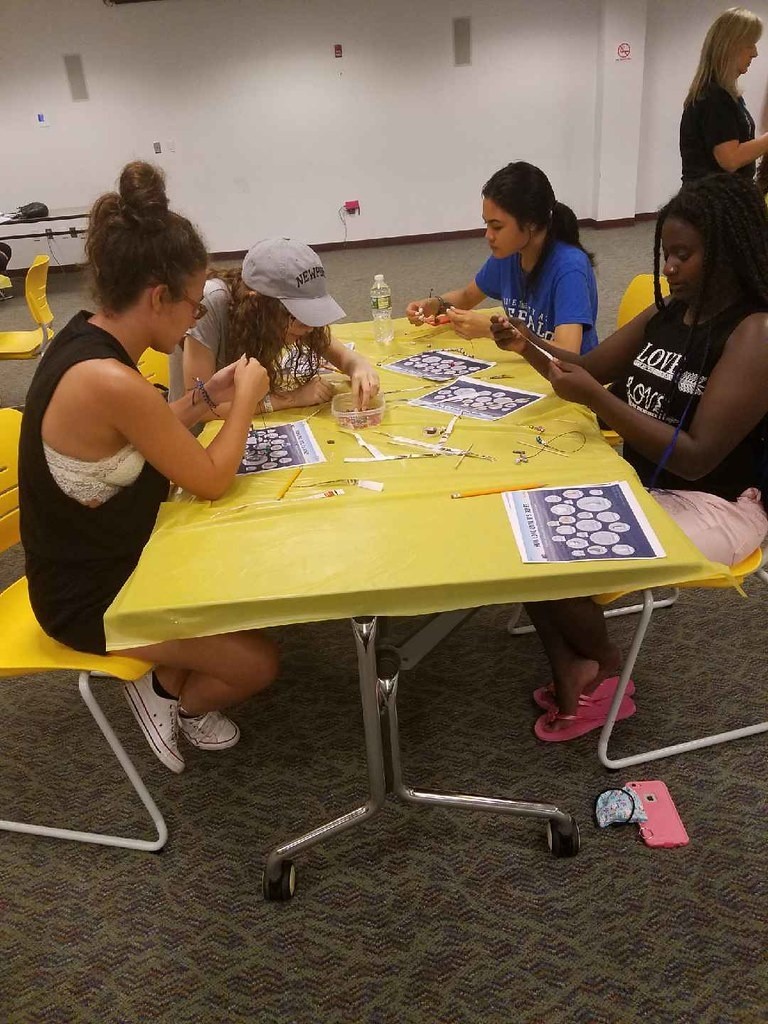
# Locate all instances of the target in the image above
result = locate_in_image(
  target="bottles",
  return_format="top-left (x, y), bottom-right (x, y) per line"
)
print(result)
top-left (369, 274), bottom-right (394, 342)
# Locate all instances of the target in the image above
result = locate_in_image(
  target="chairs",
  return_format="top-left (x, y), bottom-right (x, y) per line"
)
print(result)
top-left (0, 405), bottom-right (168, 852)
top-left (0, 254), bottom-right (54, 412)
top-left (508, 545), bottom-right (768, 769)
top-left (597, 274), bottom-right (672, 447)
top-left (137, 347), bottom-right (170, 402)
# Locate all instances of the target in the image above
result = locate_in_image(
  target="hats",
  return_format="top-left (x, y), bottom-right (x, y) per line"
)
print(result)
top-left (241, 236), bottom-right (346, 326)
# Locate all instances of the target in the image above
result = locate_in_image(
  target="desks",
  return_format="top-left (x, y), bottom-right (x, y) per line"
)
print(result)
top-left (102, 306), bottom-right (744, 902)
top-left (0, 206), bottom-right (95, 302)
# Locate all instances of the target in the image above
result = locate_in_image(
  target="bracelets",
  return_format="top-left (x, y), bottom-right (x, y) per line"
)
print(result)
top-left (435, 295), bottom-right (445, 315)
top-left (263, 393), bottom-right (274, 414)
top-left (187, 375), bottom-right (221, 418)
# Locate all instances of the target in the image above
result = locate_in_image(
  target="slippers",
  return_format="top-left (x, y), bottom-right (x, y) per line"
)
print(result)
top-left (534, 697), bottom-right (636, 741)
top-left (533, 676), bottom-right (637, 709)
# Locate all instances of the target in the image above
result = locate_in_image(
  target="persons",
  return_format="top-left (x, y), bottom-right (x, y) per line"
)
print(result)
top-left (15, 157), bottom-right (273, 771)
top-left (488, 175), bottom-right (768, 745)
top-left (406, 160), bottom-right (600, 372)
top-left (160, 237), bottom-right (380, 433)
top-left (679, 6), bottom-right (768, 193)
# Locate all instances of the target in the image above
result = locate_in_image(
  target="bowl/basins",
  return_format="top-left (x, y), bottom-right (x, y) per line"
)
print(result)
top-left (331, 391), bottom-right (385, 429)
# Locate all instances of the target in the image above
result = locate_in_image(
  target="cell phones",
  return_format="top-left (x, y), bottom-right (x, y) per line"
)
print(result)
top-left (625, 781), bottom-right (689, 847)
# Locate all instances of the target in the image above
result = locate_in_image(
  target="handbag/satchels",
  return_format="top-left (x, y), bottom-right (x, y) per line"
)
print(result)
top-left (11, 202), bottom-right (48, 219)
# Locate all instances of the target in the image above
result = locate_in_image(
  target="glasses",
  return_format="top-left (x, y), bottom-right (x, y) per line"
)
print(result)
top-left (146, 281), bottom-right (208, 319)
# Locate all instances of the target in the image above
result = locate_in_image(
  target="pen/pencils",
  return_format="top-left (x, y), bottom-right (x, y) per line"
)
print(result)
top-left (314, 361), bottom-right (346, 373)
top-left (277, 466), bottom-right (309, 499)
top-left (450, 482), bottom-right (549, 500)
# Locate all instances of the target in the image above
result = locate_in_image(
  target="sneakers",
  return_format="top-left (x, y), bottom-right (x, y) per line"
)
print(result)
top-left (177, 711), bottom-right (240, 750)
top-left (122, 669), bottom-right (185, 774)
